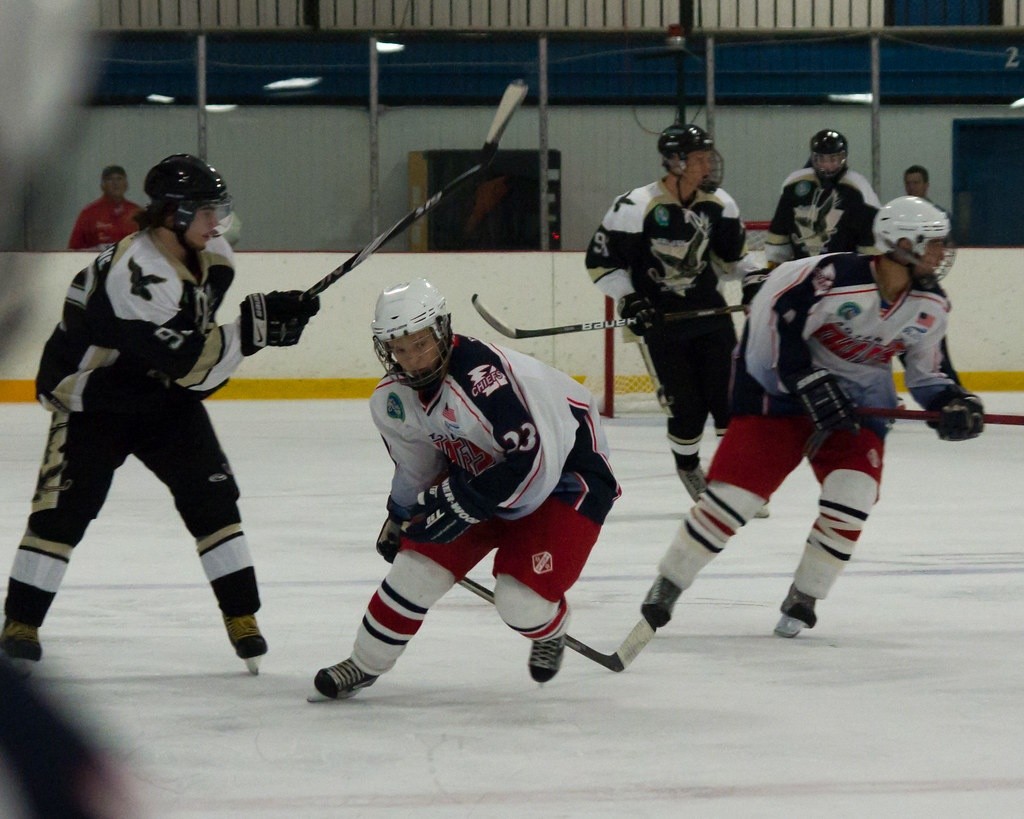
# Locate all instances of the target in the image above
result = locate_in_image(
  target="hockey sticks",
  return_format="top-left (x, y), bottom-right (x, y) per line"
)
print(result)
top-left (469, 293), bottom-right (751, 339)
top-left (859, 407), bottom-right (1022, 430)
top-left (462, 577), bottom-right (669, 672)
top-left (297, 80), bottom-right (530, 304)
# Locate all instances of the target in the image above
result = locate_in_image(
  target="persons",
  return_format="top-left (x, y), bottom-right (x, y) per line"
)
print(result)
top-left (68, 165), bottom-right (146, 251)
top-left (639, 196), bottom-right (985, 637)
top-left (308, 276), bottom-right (621, 701)
top-left (764, 129), bottom-right (883, 270)
top-left (905, 164), bottom-right (957, 247)
top-left (0, 152), bottom-right (319, 673)
top-left (586, 123), bottom-right (774, 520)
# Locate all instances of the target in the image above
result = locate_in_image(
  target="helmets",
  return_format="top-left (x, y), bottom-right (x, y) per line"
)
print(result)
top-left (144, 154), bottom-right (233, 218)
top-left (872, 195), bottom-right (952, 260)
top-left (809, 128), bottom-right (849, 178)
top-left (370, 278), bottom-right (453, 390)
top-left (657, 124), bottom-right (715, 167)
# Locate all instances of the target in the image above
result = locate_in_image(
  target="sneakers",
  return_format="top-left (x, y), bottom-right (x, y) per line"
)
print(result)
top-left (222, 609), bottom-right (269, 676)
top-left (0, 616), bottom-right (43, 679)
top-left (675, 464), bottom-right (707, 502)
top-left (774, 581), bottom-right (817, 638)
top-left (307, 657), bottom-right (379, 702)
top-left (641, 572), bottom-right (683, 631)
top-left (755, 499), bottom-right (770, 518)
top-left (528, 632), bottom-right (566, 684)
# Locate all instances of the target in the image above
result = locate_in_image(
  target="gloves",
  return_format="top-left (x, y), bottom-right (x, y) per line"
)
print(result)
top-left (924, 383), bottom-right (986, 442)
top-left (617, 292), bottom-right (660, 335)
top-left (376, 494), bottom-right (413, 565)
top-left (793, 366), bottom-right (862, 441)
top-left (240, 289), bottom-right (320, 357)
top-left (405, 475), bottom-right (491, 543)
